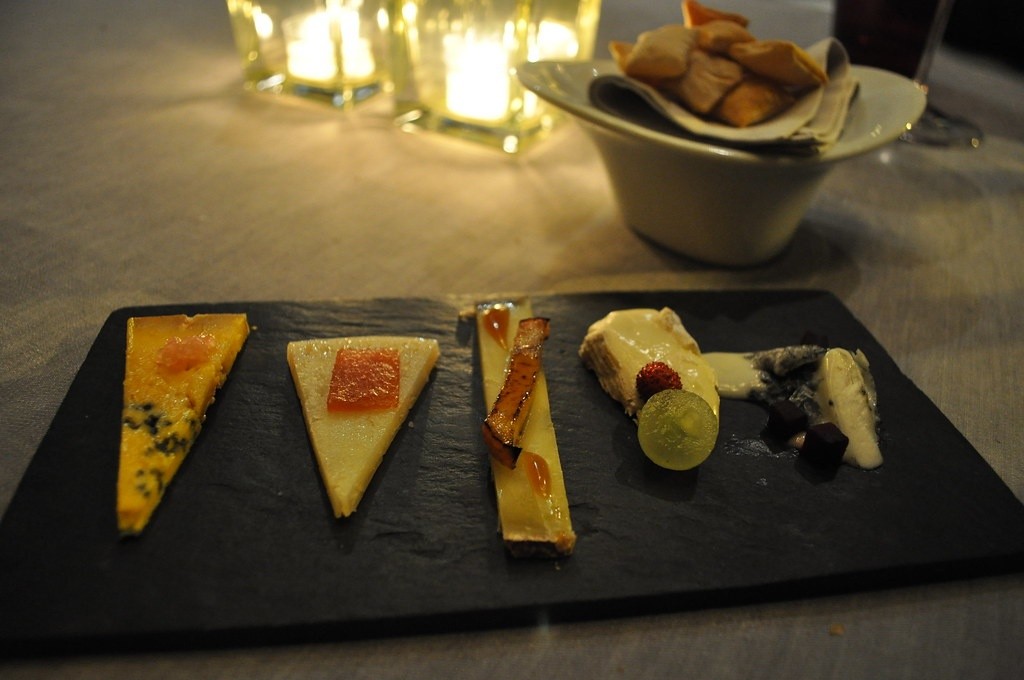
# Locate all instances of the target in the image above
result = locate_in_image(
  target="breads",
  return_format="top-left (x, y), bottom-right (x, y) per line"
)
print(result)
top-left (609, 0), bottom-right (831, 128)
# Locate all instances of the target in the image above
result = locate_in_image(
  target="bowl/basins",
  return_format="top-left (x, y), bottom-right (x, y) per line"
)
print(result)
top-left (513, 57), bottom-right (928, 263)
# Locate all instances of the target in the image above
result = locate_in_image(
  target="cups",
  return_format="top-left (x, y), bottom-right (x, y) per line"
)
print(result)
top-left (224, 0), bottom-right (386, 110)
top-left (380, 2), bottom-right (605, 155)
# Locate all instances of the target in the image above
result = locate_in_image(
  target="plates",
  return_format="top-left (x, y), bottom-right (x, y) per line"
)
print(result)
top-left (2, 289), bottom-right (1023, 661)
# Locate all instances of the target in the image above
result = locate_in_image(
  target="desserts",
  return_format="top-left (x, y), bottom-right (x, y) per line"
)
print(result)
top-left (115, 294), bottom-right (888, 558)
